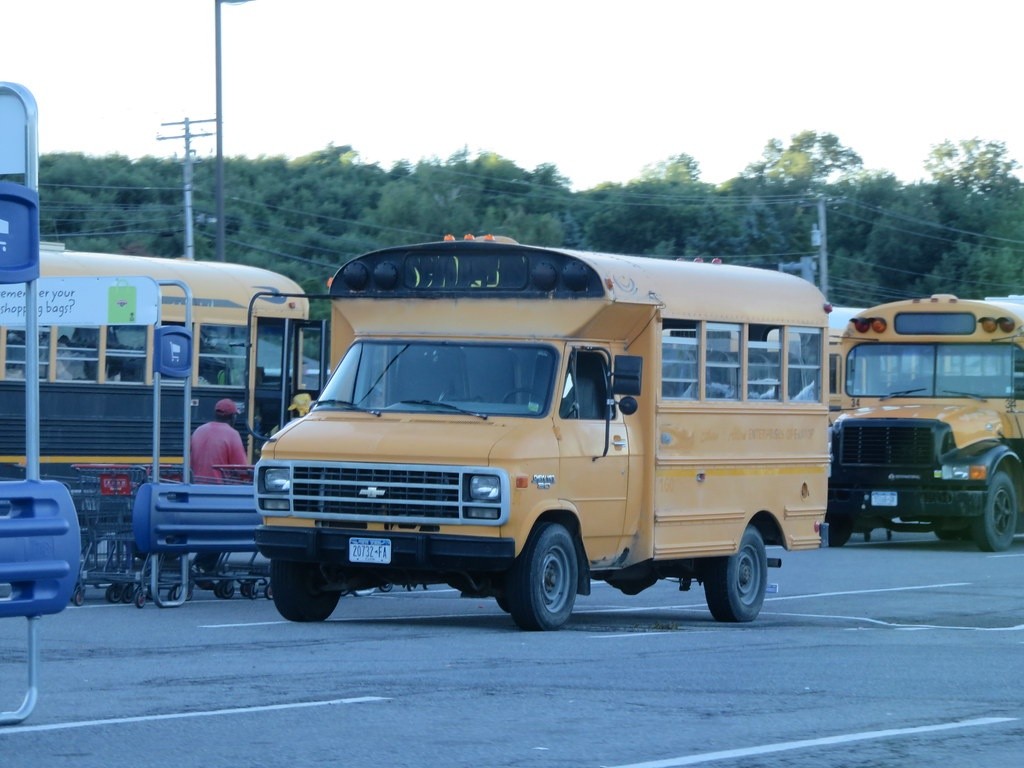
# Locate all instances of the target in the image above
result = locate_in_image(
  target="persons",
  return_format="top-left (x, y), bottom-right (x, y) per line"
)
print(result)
top-left (191, 399), bottom-right (252, 591)
top-left (270, 393), bottom-right (311, 435)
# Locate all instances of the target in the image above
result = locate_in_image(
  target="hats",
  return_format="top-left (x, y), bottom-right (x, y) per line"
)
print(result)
top-left (215, 399), bottom-right (242, 415)
top-left (288, 394), bottom-right (311, 411)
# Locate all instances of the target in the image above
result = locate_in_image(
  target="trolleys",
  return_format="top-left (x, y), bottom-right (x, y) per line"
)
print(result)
top-left (70, 460), bottom-right (429, 608)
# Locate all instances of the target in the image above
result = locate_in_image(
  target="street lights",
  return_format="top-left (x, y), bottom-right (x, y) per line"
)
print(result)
top-left (215, 0), bottom-right (251, 263)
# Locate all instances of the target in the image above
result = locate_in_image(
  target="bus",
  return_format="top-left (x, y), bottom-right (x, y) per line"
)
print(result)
top-left (0, 241), bottom-right (312, 548)
top-left (243, 232), bottom-right (834, 629)
top-left (827, 293), bottom-right (1024, 554)
